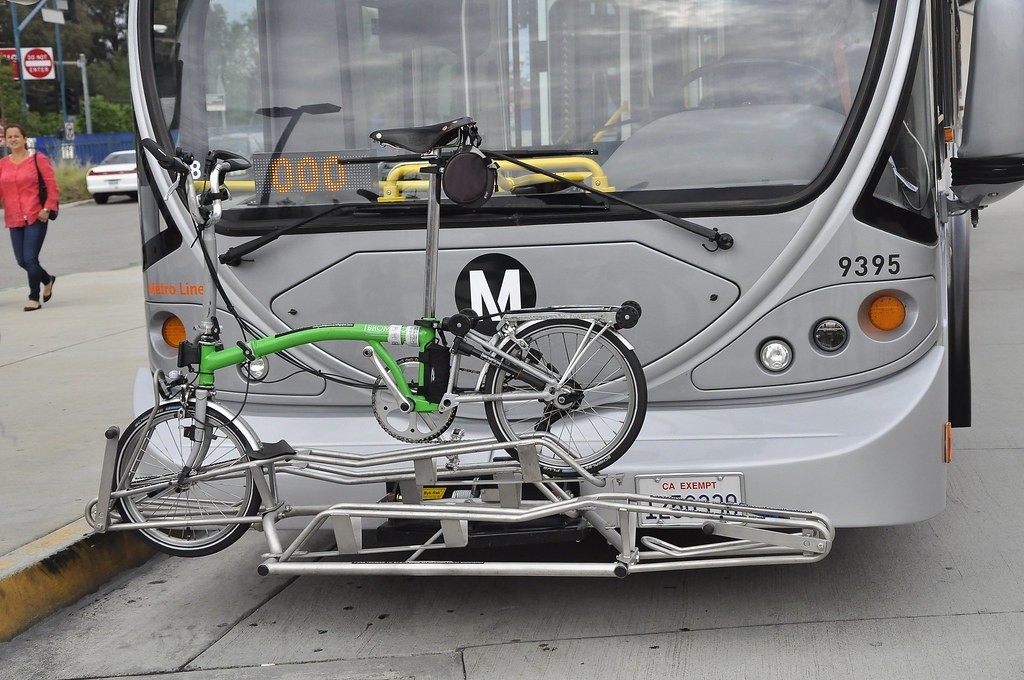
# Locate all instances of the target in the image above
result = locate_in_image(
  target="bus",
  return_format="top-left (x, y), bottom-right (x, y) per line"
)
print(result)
top-left (83, 1), bottom-right (1024, 570)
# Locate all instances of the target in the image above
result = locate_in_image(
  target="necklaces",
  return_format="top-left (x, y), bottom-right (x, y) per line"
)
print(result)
top-left (9, 150), bottom-right (30, 162)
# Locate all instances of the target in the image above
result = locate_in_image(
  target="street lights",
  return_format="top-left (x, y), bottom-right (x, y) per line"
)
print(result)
top-left (255, 102), bottom-right (341, 207)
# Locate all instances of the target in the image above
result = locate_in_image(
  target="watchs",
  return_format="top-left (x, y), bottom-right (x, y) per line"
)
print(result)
top-left (43, 208), bottom-right (51, 213)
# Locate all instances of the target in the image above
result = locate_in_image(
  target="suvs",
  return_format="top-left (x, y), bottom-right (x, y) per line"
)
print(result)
top-left (205, 134), bottom-right (261, 182)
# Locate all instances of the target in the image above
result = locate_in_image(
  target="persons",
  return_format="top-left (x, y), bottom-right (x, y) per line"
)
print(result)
top-left (1, 124), bottom-right (60, 311)
top-left (699, 0), bottom-right (874, 115)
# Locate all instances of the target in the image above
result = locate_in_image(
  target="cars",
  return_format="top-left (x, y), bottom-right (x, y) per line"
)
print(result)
top-left (87, 150), bottom-right (139, 203)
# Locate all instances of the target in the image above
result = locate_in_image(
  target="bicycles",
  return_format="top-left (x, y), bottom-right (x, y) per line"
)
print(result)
top-left (113, 116), bottom-right (648, 558)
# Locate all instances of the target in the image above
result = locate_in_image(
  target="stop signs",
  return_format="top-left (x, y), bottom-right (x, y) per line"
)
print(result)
top-left (21, 46), bottom-right (55, 80)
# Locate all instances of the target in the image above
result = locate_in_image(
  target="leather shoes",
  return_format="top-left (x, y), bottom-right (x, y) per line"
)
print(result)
top-left (24, 304), bottom-right (41, 311)
top-left (43, 275), bottom-right (56, 303)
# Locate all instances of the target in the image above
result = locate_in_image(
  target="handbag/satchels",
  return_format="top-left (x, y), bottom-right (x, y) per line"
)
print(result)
top-left (34, 152), bottom-right (59, 221)
